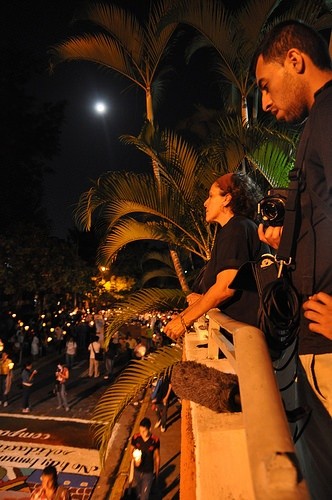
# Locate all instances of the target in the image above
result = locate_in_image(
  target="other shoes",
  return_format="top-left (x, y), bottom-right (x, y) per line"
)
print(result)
top-left (103, 375), bottom-right (109, 379)
top-left (155, 419), bottom-right (161, 428)
top-left (57, 405), bottom-right (61, 410)
top-left (66, 407), bottom-right (70, 411)
top-left (161, 426), bottom-right (165, 433)
top-left (23, 407), bottom-right (30, 413)
top-left (4, 401), bottom-right (7, 407)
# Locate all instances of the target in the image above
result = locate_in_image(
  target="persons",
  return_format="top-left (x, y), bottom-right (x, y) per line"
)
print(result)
top-left (253, 19), bottom-right (332, 414)
top-left (0, 230), bottom-right (181, 500)
top-left (164, 171), bottom-right (297, 441)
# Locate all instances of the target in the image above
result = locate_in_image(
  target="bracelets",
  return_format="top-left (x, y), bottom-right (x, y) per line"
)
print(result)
top-left (179, 312), bottom-right (191, 335)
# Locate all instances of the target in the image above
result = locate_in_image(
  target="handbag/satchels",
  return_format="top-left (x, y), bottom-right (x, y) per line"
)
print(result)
top-left (95, 352), bottom-right (103, 361)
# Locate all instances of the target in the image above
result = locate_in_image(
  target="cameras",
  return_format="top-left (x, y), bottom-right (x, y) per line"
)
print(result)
top-left (258, 188), bottom-right (290, 226)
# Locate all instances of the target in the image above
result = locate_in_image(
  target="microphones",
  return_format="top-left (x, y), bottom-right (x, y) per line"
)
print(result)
top-left (172, 361), bottom-right (242, 414)
